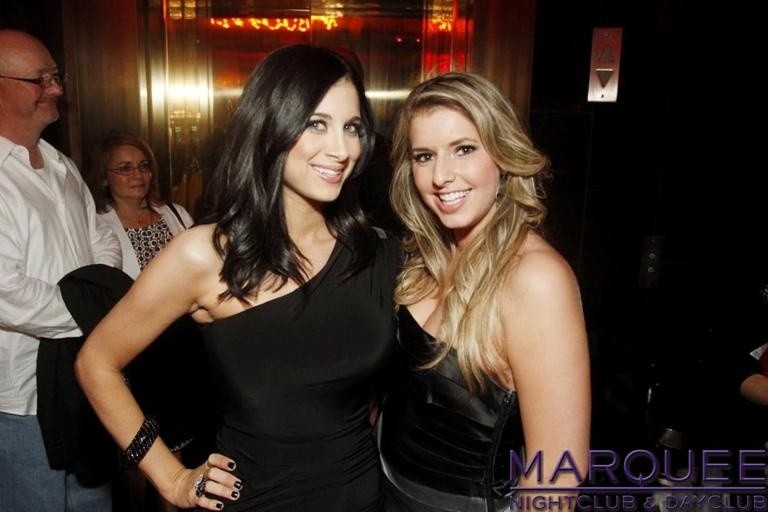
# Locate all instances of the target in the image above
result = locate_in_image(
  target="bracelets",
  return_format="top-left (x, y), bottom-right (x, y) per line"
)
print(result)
top-left (120, 414), bottom-right (160, 466)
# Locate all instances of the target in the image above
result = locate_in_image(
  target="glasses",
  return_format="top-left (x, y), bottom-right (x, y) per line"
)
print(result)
top-left (0, 70), bottom-right (69, 89)
top-left (105, 163), bottom-right (153, 176)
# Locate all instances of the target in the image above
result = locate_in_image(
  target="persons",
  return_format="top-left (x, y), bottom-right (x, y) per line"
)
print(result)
top-left (722, 284), bottom-right (767, 407)
top-left (362, 71), bottom-right (592, 512)
top-left (0, 30), bottom-right (121, 510)
top-left (92, 131), bottom-right (198, 282)
top-left (72, 44), bottom-right (401, 512)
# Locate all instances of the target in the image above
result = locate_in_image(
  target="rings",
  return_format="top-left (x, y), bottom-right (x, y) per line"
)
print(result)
top-left (194, 475), bottom-right (205, 497)
